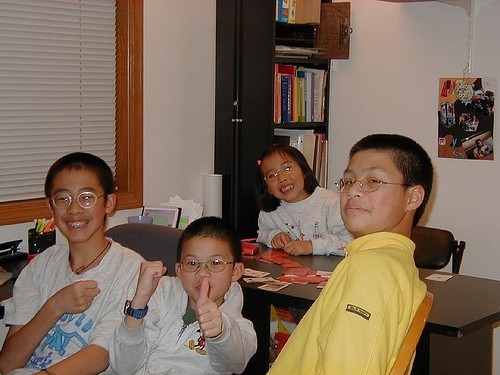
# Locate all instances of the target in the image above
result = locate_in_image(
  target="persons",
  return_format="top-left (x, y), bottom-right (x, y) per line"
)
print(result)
top-left (255, 142), bottom-right (355, 258)
top-left (0, 155), bottom-right (147, 375)
top-left (266, 134), bottom-right (434, 375)
top-left (110, 217), bottom-right (257, 375)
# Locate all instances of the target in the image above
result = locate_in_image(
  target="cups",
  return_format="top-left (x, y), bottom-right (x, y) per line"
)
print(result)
top-left (28, 228), bottom-right (56, 255)
top-left (127, 216), bottom-right (153, 224)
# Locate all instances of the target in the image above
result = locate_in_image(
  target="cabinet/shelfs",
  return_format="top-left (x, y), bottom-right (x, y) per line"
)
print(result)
top-left (214, 0), bottom-right (334, 264)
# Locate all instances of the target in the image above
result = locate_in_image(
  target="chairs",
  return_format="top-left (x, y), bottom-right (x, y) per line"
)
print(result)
top-left (103, 224), bottom-right (184, 277)
top-left (410, 225), bottom-right (466, 274)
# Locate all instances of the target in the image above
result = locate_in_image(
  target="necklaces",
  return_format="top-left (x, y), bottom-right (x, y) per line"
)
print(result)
top-left (74, 240), bottom-right (112, 275)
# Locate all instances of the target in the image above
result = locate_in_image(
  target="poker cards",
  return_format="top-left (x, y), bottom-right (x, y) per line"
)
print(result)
top-left (241, 248), bottom-right (333, 292)
top-left (426, 272), bottom-right (453, 282)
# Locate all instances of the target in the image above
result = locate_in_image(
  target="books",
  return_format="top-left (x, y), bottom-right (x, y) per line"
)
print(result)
top-left (274, 0), bottom-right (329, 189)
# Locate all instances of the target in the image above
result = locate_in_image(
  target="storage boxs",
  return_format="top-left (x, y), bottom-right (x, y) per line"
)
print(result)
top-left (241, 242), bottom-right (260, 255)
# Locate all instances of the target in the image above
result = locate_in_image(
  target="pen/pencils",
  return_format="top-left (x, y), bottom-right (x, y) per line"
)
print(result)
top-left (32, 216), bottom-right (57, 233)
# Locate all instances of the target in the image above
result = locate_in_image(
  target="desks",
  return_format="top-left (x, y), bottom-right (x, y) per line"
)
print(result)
top-left (236, 242), bottom-right (500, 375)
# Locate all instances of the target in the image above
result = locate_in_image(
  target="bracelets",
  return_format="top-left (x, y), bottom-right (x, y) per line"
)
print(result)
top-left (124, 299), bottom-right (148, 319)
top-left (41, 368), bottom-right (50, 375)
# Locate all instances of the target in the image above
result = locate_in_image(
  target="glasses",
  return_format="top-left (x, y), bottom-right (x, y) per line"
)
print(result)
top-left (49, 192), bottom-right (105, 210)
top-left (179, 258), bottom-right (234, 273)
top-left (263, 162), bottom-right (298, 183)
top-left (334, 176), bottom-right (404, 193)
